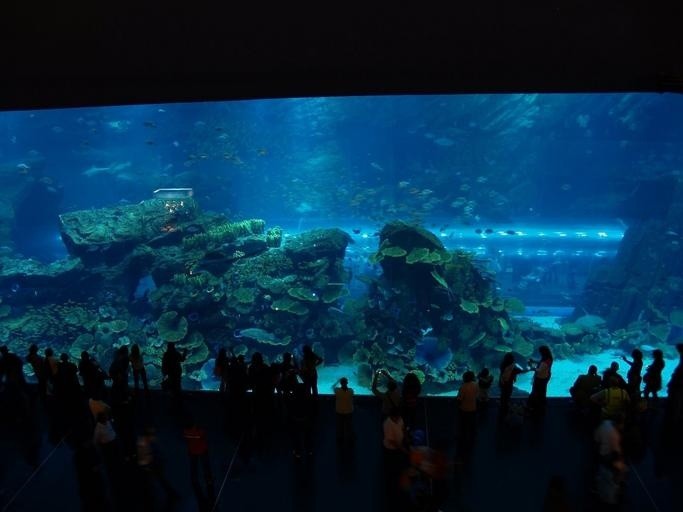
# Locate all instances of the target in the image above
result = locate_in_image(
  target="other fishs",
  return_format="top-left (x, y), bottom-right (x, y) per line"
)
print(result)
top-left (0, 94), bottom-right (683, 392)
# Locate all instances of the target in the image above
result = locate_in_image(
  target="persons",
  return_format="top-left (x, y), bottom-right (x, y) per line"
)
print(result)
top-left (0, 342), bottom-right (683, 512)
